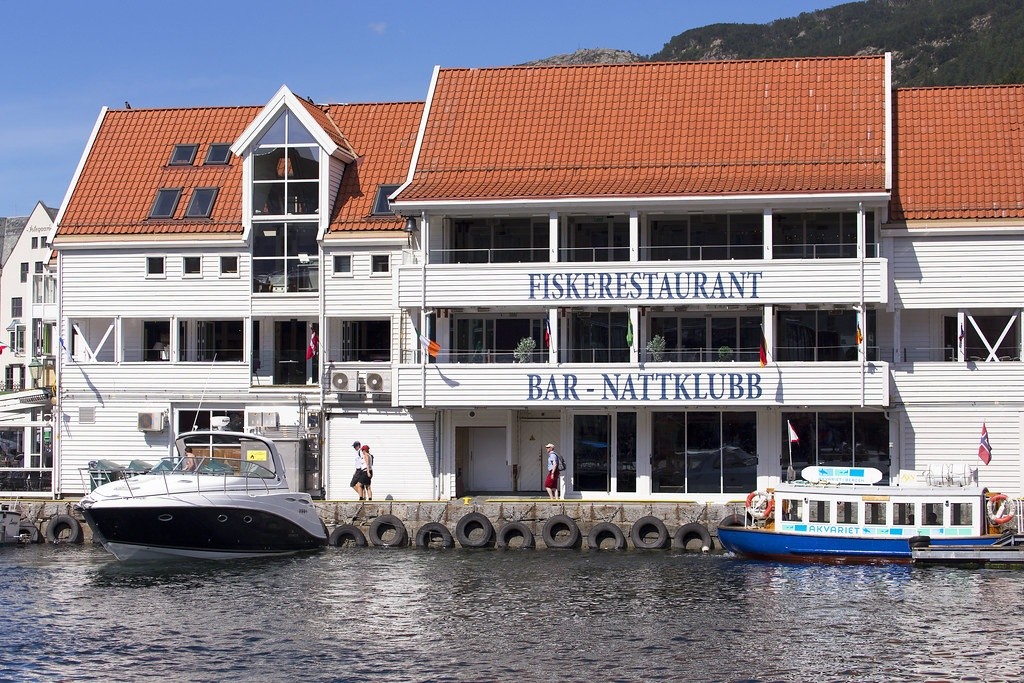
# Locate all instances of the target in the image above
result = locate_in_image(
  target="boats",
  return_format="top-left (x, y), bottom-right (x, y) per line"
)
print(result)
top-left (74, 353), bottom-right (330, 562)
top-left (713, 420), bottom-right (1024, 564)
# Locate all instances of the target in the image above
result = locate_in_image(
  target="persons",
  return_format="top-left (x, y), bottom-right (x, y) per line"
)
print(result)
top-left (544, 443), bottom-right (560, 499)
top-left (908, 513), bottom-right (941, 524)
top-left (350, 441), bottom-right (372, 500)
top-left (185, 447), bottom-right (196, 471)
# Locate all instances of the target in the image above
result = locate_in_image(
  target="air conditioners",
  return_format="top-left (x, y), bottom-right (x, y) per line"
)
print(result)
top-left (366, 372), bottom-right (392, 393)
top-left (137, 411), bottom-right (164, 431)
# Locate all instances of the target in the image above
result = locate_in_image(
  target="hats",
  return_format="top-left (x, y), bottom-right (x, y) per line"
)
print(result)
top-left (352, 441), bottom-right (361, 446)
top-left (361, 444), bottom-right (370, 451)
top-left (545, 443), bottom-right (555, 448)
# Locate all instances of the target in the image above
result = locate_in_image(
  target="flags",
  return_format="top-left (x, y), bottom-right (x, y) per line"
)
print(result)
top-left (305, 329), bottom-right (318, 360)
top-left (760, 331), bottom-right (767, 367)
top-left (0, 342), bottom-right (8, 355)
top-left (627, 319), bottom-right (633, 346)
top-left (790, 424), bottom-right (799, 445)
top-left (545, 319), bottom-right (551, 347)
top-left (979, 423), bottom-right (992, 465)
top-left (959, 325), bottom-right (965, 341)
top-left (415, 328), bottom-right (441, 358)
top-left (856, 324), bottom-right (863, 344)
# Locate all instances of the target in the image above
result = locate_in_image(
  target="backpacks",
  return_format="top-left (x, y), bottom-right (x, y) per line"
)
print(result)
top-left (549, 452), bottom-right (567, 471)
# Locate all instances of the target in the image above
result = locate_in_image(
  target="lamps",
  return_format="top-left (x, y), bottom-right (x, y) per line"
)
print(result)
top-left (28, 356), bottom-right (57, 400)
top-left (329, 370), bottom-right (359, 392)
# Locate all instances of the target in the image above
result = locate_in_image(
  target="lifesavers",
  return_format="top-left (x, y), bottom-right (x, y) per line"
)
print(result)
top-left (988, 494), bottom-right (1015, 524)
top-left (909, 536), bottom-right (931, 548)
top-left (19, 521), bottom-right (39, 544)
top-left (46, 514), bottom-right (81, 545)
top-left (327, 513), bottom-right (753, 553)
top-left (746, 490), bottom-right (772, 519)
